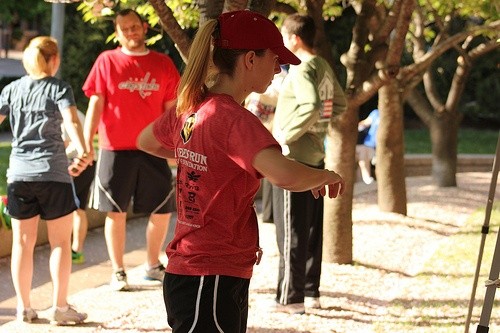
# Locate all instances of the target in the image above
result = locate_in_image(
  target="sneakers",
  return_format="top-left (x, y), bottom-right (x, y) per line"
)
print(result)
top-left (112, 271), bottom-right (130, 290)
top-left (50, 307), bottom-right (86, 327)
top-left (17, 308), bottom-right (36, 322)
top-left (143, 264), bottom-right (167, 281)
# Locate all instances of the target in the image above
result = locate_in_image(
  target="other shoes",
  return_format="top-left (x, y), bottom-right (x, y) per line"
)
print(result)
top-left (304, 296), bottom-right (321, 308)
top-left (72, 250), bottom-right (84, 264)
top-left (267, 299), bottom-right (304, 315)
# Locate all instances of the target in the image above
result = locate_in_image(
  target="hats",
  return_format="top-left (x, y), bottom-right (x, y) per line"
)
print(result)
top-left (211, 9), bottom-right (301, 66)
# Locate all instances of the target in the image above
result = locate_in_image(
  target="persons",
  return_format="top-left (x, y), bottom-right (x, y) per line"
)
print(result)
top-left (0, 107), bottom-right (96, 264)
top-left (355, 102), bottom-right (380, 184)
top-left (244, 61), bottom-right (289, 225)
top-left (135, 11), bottom-right (345, 333)
top-left (0, 35), bottom-right (88, 324)
top-left (265, 13), bottom-right (348, 316)
top-left (82, 8), bottom-right (184, 292)
